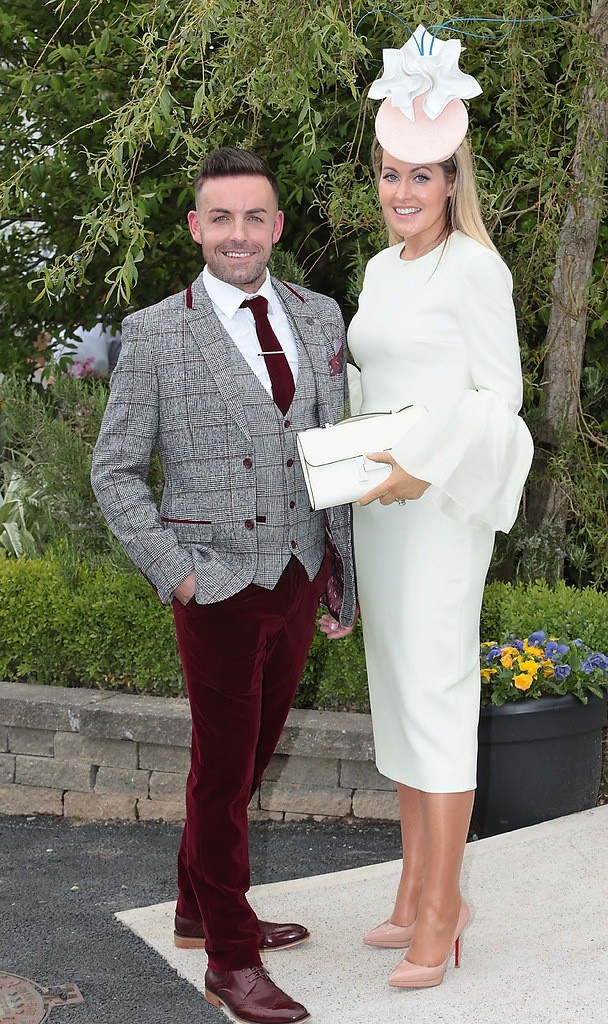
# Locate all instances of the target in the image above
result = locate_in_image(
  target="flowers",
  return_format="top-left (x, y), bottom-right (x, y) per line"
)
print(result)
top-left (479, 626), bottom-right (608, 709)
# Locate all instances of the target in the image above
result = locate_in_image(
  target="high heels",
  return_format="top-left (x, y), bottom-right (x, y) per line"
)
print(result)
top-left (386, 895), bottom-right (471, 987)
top-left (363, 916), bottom-right (417, 949)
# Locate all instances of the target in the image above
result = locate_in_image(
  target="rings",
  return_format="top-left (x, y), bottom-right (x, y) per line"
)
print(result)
top-left (396, 498), bottom-right (412, 510)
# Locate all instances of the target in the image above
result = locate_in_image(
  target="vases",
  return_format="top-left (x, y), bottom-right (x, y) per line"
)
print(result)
top-left (470, 684), bottom-right (607, 839)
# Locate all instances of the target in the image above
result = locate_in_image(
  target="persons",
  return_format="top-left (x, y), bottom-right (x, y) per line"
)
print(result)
top-left (344, 24), bottom-right (536, 990)
top-left (92, 146), bottom-right (358, 1024)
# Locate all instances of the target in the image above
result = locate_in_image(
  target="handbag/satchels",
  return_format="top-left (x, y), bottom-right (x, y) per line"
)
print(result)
top-left (295, 402), bottom-right (433, 512)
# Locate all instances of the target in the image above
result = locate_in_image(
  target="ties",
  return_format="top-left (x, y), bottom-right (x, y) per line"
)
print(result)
top-left (240, 296), bottom-right (296, 416)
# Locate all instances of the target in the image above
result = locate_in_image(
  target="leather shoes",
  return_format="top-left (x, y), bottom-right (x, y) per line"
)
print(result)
top-left (172, 899), bottom-right (311, 953)
top-left (203, 963), bottom-right (312, 1024)
